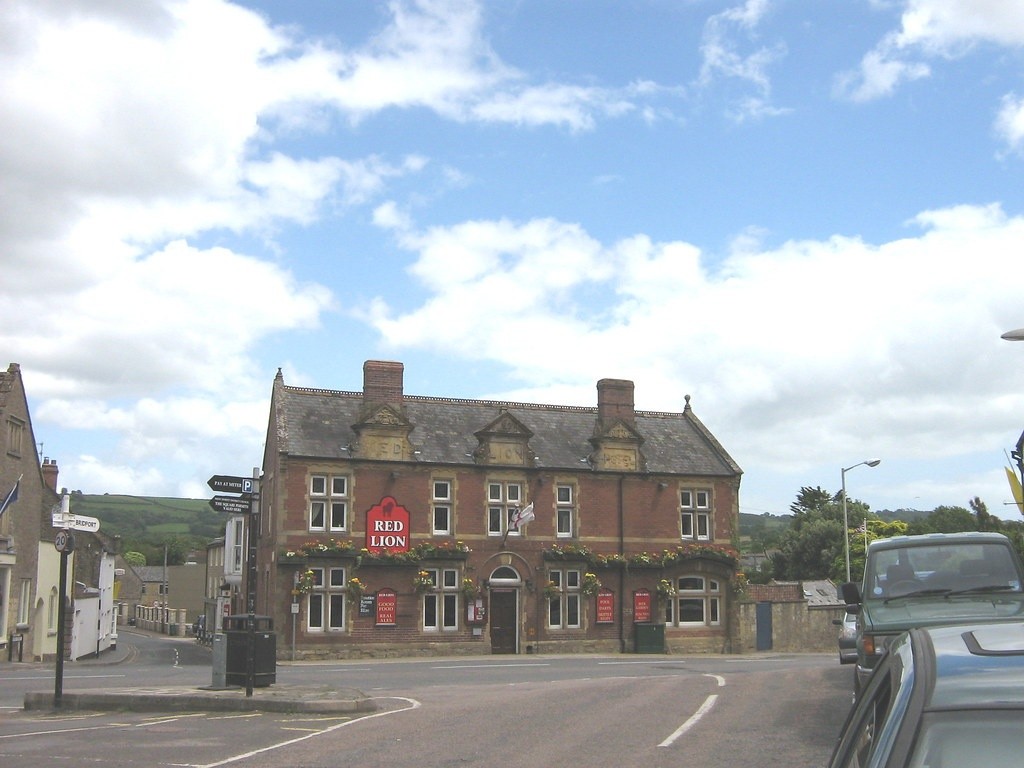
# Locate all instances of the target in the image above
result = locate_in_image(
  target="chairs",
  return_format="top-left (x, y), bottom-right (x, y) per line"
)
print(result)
top-left (886, 563), bottom-right (914, 593)
top-left (958, 557), bottom-right (987, 576)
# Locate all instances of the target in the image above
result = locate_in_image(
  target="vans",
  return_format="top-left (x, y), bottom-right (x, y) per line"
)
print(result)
top-left (842, 532), bottom-right (1024, 708)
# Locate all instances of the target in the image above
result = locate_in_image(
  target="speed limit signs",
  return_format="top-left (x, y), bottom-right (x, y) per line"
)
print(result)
top-left (54, 530), bottom-right (68, 552)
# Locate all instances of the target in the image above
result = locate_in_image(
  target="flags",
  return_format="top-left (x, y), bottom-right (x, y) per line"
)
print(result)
top-left (508, 501), bottom-right (536, 531)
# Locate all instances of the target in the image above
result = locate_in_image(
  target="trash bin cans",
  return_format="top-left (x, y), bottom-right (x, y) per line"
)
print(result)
top-left (222, 613), bottom-right (277, 687)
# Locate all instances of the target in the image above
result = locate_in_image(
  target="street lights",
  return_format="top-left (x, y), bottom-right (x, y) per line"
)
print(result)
top-left (841, 458), bottom-right (881, 582)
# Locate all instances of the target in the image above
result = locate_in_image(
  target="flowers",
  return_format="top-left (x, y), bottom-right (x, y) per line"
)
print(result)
top-left (278, 536), bottom-right (479, 600)
top-left (542, 542), bottom-right (739, 601)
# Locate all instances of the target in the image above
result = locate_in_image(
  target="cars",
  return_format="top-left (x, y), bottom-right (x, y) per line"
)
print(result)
top-left (827, 621), bottom-right (1024, 768)
top-left (832, 610), bottom-right (860, 664)
